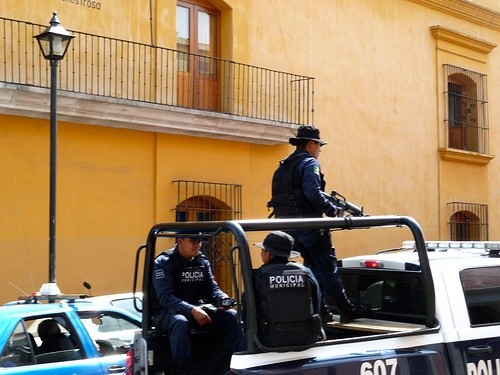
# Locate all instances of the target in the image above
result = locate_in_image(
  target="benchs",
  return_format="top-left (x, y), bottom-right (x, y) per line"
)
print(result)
top-left (326, 318), bottom-right (427, 333)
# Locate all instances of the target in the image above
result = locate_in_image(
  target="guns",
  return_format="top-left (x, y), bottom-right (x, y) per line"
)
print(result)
top-left (319, 191), bottom-right (370, 217)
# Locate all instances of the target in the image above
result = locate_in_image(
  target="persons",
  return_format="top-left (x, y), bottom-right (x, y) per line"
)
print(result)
top-left (271, 125), bottom-right (370, 323)
top-left (151, 230), bottom-right (239, 375)
top-left (241, 230), bottom-right (322, 348)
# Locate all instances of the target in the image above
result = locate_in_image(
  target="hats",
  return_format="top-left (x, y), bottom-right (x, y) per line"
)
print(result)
top-left (175, 231), bottom-right (208, 242)
top-left (253, 231), bottom-right (300, 259)
top-left (289, 126), bottom-right (327, 147)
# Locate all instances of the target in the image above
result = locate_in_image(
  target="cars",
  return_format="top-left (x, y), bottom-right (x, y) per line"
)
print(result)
top-left (22, 290), bottom-right (143, 354)
top-left (0, 283), bottom-right (150, 375)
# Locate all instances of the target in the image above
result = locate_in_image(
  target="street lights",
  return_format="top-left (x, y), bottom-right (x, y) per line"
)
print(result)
top-left (33, 10), bottom-right (75, 303)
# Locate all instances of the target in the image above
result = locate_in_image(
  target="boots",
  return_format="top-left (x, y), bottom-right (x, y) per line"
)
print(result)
top-left (340, 298), bottom-right (368, 324)
top-left (321, 304), bottom-right (333, 322)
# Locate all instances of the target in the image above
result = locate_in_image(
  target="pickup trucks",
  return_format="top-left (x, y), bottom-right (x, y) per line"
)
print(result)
top-left (129, 216), bottom-right (499, 375)
